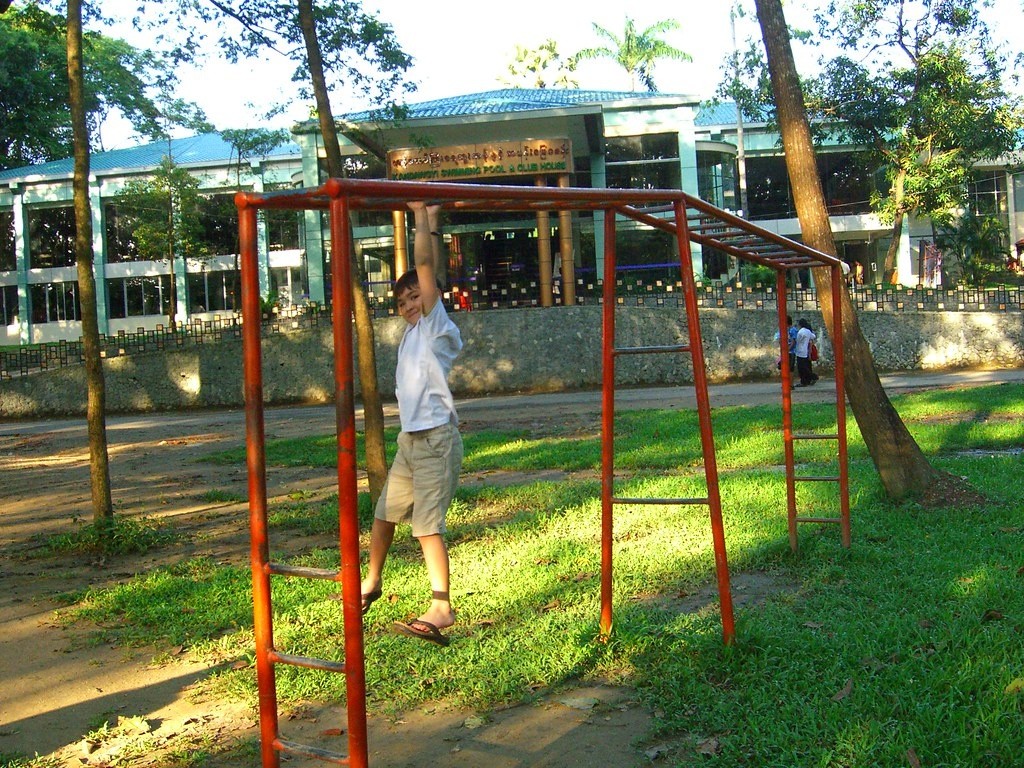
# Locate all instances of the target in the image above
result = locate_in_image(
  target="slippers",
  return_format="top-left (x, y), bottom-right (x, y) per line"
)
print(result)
top-left (392, 619), bottom-right (449, 647)
top-left (341, 590), bottom-right (381, 614)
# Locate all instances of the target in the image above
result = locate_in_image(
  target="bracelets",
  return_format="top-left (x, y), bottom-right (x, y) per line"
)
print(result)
top-left (431, 231), bottom-right (440, 236)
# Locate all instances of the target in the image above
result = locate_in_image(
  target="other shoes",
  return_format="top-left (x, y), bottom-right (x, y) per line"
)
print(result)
top-left (811, 377), bottom-right (819, 385)
top-left (781, 385), bottom-right (795, 390)
top-left (795, 383), bottom-right (807, 387)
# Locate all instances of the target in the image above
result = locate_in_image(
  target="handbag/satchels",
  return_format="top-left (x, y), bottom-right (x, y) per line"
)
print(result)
top-left (811, 344), bottom-right (818, 361)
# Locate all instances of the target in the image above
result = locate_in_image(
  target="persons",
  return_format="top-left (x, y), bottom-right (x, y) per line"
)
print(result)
top-left (360, 201), bottom-right (463, 647)
top-left (1006, 254), bottom-right (1017, 272)
top-left (840, 257), bottom-right (864, 286)
top-left (774, 315), bottom-right (819, 389)
top-left (1018, 249), bottom-right (1024, 271)
